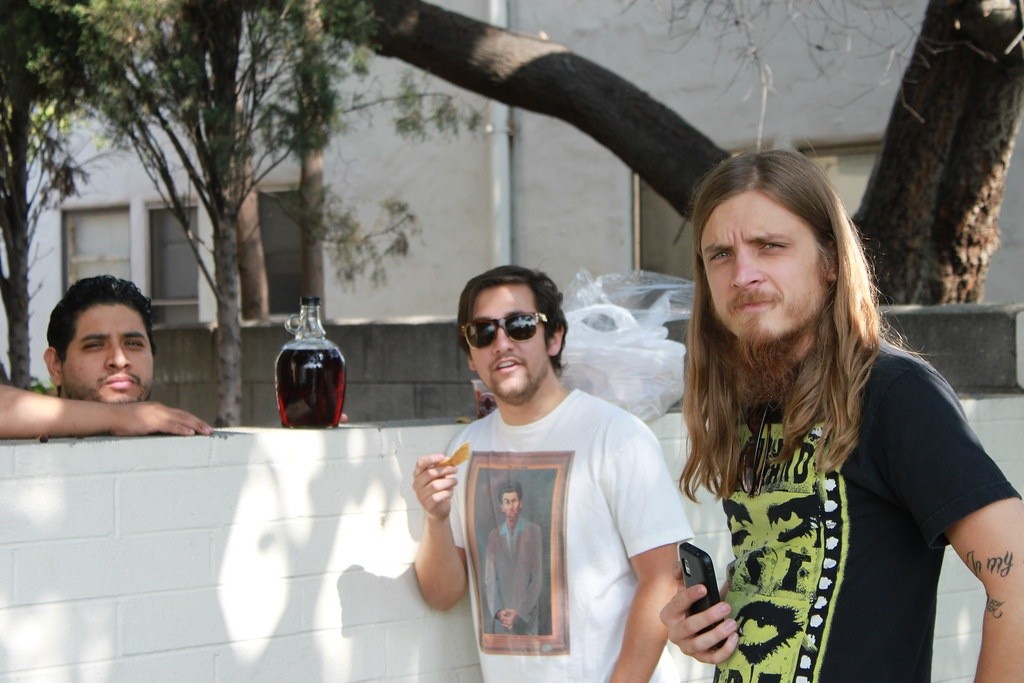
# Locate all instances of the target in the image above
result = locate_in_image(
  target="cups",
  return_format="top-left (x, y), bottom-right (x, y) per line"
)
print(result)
top-left (472, 379), bottom-right (497, 419)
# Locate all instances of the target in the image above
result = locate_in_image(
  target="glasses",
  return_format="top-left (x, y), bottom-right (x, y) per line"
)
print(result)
top-left (460, 312), bottom-right (548, 351)
top-left (739, 394), bottom-right (774, 498)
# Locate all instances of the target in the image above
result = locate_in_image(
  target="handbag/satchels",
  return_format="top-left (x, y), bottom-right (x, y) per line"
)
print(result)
top-left (561, 303), bottom-right (687, 422)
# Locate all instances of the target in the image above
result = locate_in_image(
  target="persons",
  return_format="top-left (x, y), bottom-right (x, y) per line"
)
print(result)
top-left (660, 147), bottom-right (1024, 683)
top-left (0, 275), bottom-right (214, 437)
top-left (413, 265), bottom-right (695, 683)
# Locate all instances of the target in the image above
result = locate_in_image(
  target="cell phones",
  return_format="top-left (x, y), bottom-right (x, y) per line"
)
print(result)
top-left (680, 542), bottom-right (727, 649)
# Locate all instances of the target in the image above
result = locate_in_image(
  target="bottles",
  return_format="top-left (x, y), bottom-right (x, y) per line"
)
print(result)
top-left (275, 296), bottom-right (347, 428)
